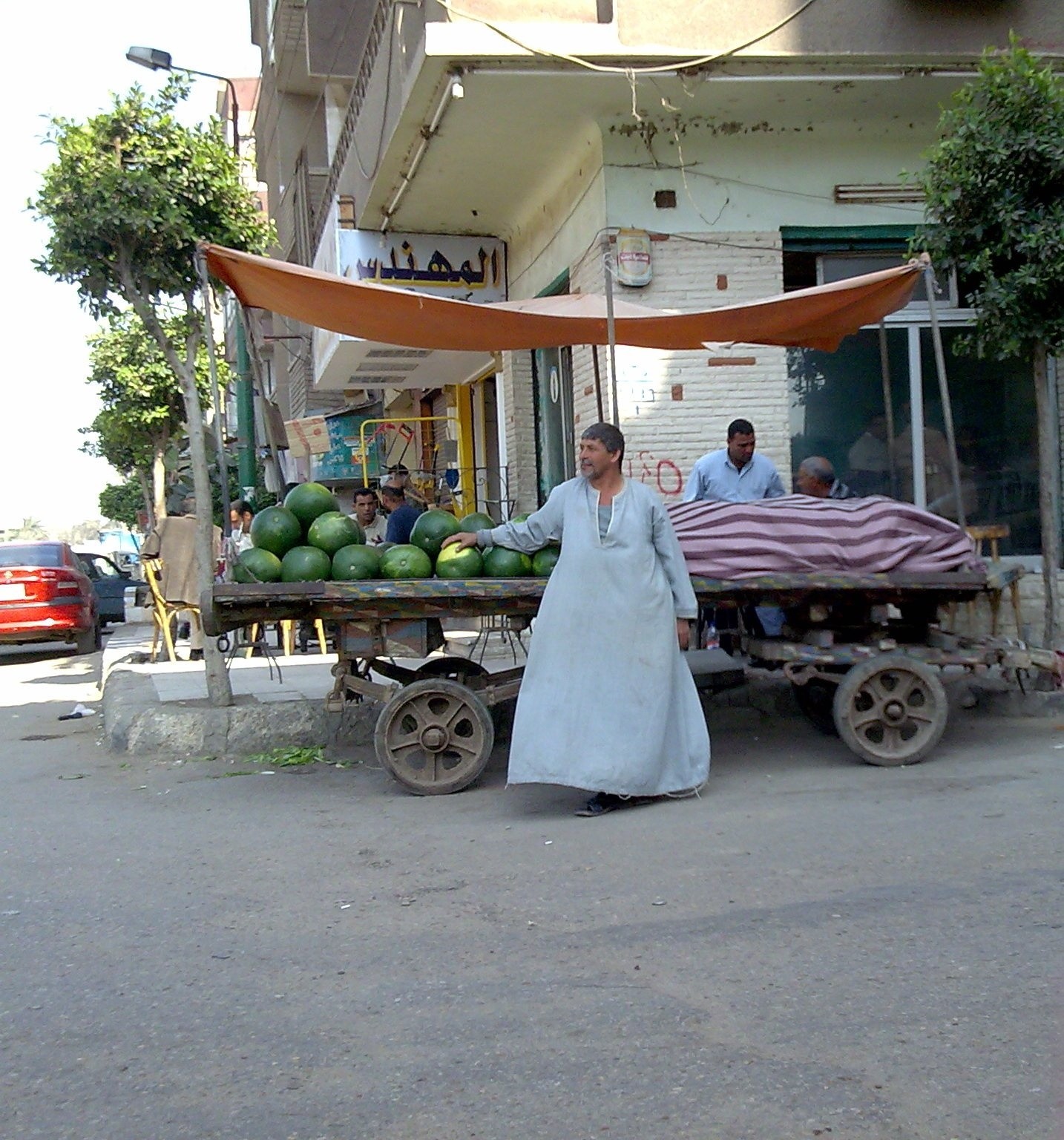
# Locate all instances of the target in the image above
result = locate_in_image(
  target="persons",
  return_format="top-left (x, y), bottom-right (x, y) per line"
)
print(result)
top-left (849, 414), bottom-right (892, 472)
top-left (440, 423), bottom-right (711, 816)
top-left (796, 457), bottom-right (859, 499)
top-left (346, 488), bottom-right (388, 544)
top-left (229, 500), bottom-right (257, 555)
top-left (894, 400), bottom-right (978, 519)
top-left (140, 492), bottom-right (223, 661)
top-left (382, 480), bottom-right (422, 544)
top-left (684, 419), bottom-right (785, 503)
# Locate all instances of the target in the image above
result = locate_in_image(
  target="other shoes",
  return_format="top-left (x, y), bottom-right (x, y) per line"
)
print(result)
top-left (630, 797), bottom-right (652, 806)
top-left (572, 794), bottom-right (616, 816)
top-left (179, 629), bottom-right (190, 639)
top-left (189, 649), bottom-right (204, 660)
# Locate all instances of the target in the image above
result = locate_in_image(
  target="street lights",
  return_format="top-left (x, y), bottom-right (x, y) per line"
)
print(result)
top-left (126, 46), bottom-right (259, 514)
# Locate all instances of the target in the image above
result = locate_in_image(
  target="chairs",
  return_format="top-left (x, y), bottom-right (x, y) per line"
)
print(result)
top-left (949, 524), bottom-right (1024, 637)
top-left (140, 557), bottom-right (326, 663)
top-left (467, 615), bottom-right (528, 666)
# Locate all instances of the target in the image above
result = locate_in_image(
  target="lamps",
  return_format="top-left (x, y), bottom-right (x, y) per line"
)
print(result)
top-left (835, 182), bottom-right (931, 205)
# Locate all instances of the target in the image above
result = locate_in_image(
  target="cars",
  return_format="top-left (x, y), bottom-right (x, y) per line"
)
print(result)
top-left (0, 543), bottom-right (102, 654)
top-left (75, 551), bottom-right (151, 628)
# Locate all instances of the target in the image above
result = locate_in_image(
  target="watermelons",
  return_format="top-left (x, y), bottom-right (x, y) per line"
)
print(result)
top-left (231, 479), bottom-right (560, 584)
